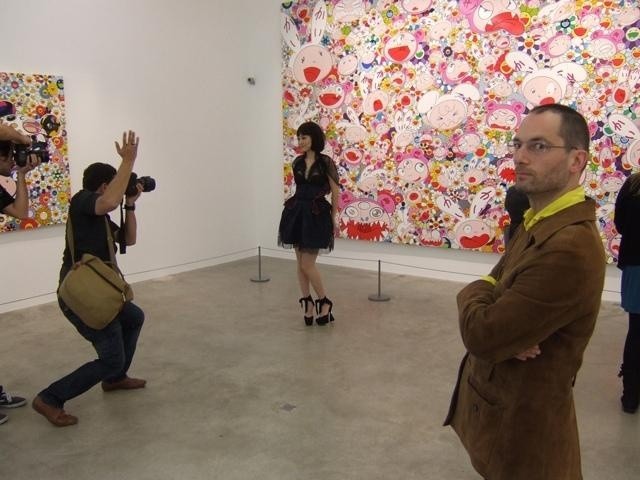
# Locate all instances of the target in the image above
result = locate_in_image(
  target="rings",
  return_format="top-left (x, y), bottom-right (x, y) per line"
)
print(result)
top-left (132, 143), bottom-right (135, 145)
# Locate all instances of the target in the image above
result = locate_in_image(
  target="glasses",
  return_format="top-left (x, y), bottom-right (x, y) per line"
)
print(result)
top-left (505, 137), bottom-right (579, 156)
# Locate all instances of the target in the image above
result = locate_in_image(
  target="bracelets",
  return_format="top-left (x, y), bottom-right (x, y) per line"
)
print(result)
top-left (124, 204), bottom-right (135, 210)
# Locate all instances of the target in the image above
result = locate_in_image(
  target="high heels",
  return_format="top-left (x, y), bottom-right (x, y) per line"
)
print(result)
top-left (315, 295), bottom-right (335, 326)
top-left (299, 293), bottom-right (314, 326)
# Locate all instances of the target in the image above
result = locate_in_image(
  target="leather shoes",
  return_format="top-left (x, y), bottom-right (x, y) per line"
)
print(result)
top-left (100, 374), bottom-right (147, 393)
top-left (31, 394), bottom-right (78, 427)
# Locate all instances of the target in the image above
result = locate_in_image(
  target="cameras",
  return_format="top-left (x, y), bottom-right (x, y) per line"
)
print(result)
top-left (126, 172), bottom-right (155, 197)
top-left (14, 145), bottom-right (49, 167)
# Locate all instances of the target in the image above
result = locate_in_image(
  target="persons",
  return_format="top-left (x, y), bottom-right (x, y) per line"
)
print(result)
top-left (32, 127), bottom-right (145, 429)
top-left (441, 101), bottom-right (608, 480)
top-left (501, 184), bottom-right (530, 251)
top-left (613, 172), bottom-right (639, 415)
top-left (276, 122), bottom-right (343, 326)
top-left (1, 121), bottom-right (42, 426)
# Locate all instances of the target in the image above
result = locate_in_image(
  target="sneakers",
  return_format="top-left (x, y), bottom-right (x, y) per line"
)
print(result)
top-left (0, 385), bottom-right (27, 426)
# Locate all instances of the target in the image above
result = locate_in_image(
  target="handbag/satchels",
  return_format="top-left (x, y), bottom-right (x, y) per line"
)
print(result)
top-left (56, 188), bottom-right (134, 331)
